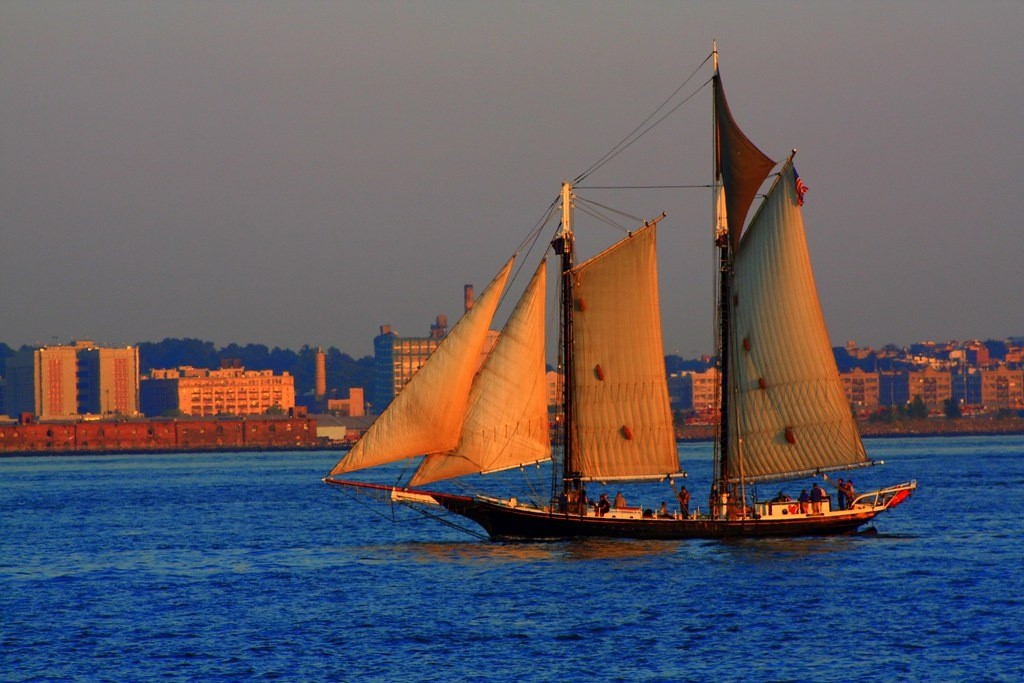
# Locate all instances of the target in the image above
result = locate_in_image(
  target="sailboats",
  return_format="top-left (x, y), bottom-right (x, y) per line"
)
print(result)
top-left (319, 35), bottom-right (919, 541)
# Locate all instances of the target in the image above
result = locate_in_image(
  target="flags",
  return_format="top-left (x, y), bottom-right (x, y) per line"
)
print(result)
top-left (792, 159), bottom-right (809, 206)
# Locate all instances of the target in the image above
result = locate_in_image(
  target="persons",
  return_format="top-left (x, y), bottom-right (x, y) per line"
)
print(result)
top-left (580, 483), bottom-right (719, 520)
top-left (779, 478), bottom-right (854, 516)
